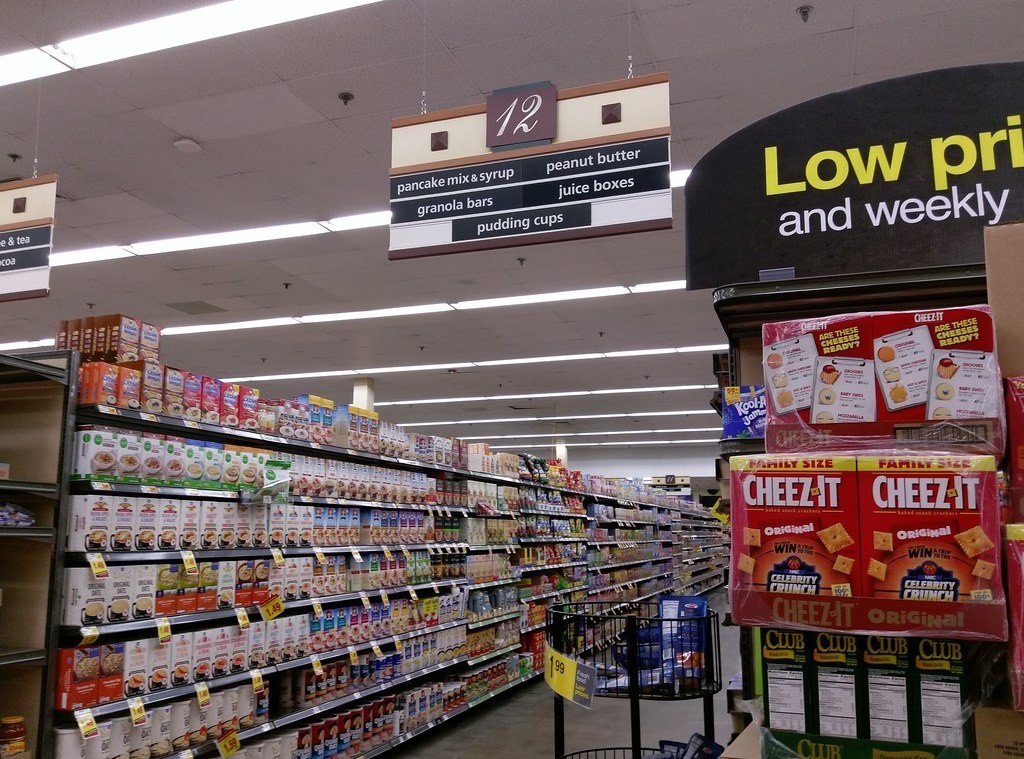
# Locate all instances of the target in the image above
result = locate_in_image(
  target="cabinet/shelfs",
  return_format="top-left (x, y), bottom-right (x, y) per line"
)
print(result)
top-left (674, 508), bottom-right (725, 596)
top-left (13, 349), bottom-right (674, 759)
top-left (0, 354), bottom-right (70, 759)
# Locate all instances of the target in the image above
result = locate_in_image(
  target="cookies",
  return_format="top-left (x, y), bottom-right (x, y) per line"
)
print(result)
top-left (972, 559), bottom-right (996, 579)
top-left (737, 553), bottom-right (755, 575)
top-left (743, 527), bottom-right (760, 546)
top-left (816, 522), bottom-right (854, 554)
top-left (954, 526), bottom-right (995, 558)
top-left (867, 558), bottom-right (887, 581)
top-left (873, 531), bottom-right (893, 551)
top-left (832, 555), bottom-right (855, 574)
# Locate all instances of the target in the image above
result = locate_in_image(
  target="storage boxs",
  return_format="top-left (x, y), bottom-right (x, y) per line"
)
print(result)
top-left (54, 394), bottom-right (545, 759)
top-left (79, 363), bottom-right (91, 404)
top-left (119, 366), bottom-right (143, 410)
top-left (91, 362), bottom-right (120, 406)
top-left (131, 360), bottom-right (165, 415)
top-left (761, 304), bottom-right (1007, 461)
top-left (66, 318), bottom-right (82, 351)
top-left (220, 382), bottom-right (241, 428)
top-left (721, 384), bottom-right (766, 438)
top-left (201, 375), bottom-right (221, 426)
top-left (728, 453), bottom-right (1024, 759)
top-left (183, 371), bottom-right (203, 422)
top-left (983, 220), bottom-right (1024, 378)
top-left (92, 314), bottom-right (108, 362)
top-left (138, 320), bottom-right (160, 364)
top-left (105, 314), bottom-right (142, 364)
top-left (55, 320), bottom-right (69, 350)
top-left (163, 365), bottom-right (186, 420)
top-left (239, 385), bottom-right (260, 431)
top-left (80, 317), bottom-right (95, 362)
